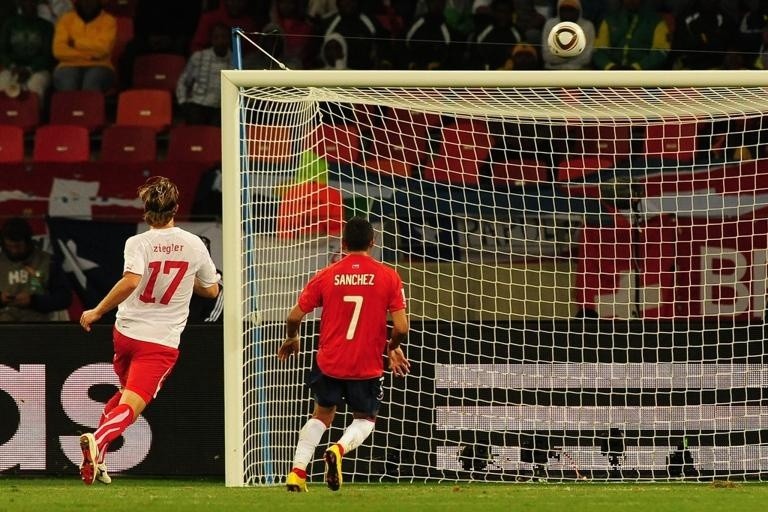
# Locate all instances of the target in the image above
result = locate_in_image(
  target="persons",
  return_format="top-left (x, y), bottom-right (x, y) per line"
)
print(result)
top-left (77, 174), bottom-right (220, 487)
top-left (275, 215), bottom-right (413, 492)
top-left (2, 0), bottom-right (768, 323)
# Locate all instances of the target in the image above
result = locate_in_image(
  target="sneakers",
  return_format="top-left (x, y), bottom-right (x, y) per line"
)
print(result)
top-left (323, 443), bottom-right (343, 491)
top-left (286, 472), bottom-right (309, 492)
top-left (96, 460), bottom-right (112, 484)
top-left (78, 432), bottom-right (100, 486)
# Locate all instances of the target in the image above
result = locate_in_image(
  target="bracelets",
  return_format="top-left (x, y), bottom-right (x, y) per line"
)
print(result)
top-left (287, 333), bottom-right (299, 341)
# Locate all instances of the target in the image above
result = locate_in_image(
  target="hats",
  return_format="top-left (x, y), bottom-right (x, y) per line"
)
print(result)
top-left (512, 42), bottom-right (537, 61)
top-left (559, 0), bottom-right (580, 10)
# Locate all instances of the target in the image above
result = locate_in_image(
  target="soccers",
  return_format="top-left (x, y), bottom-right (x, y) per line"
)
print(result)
top-left (547, 21), bottom-right (586, 57)
